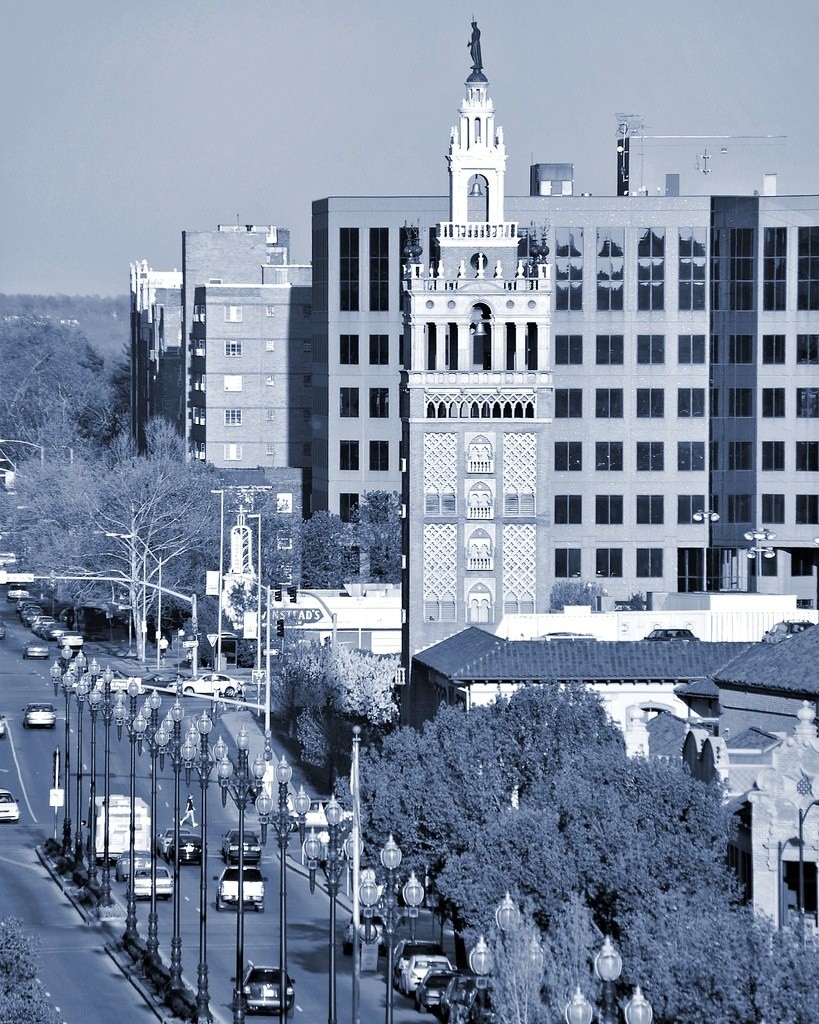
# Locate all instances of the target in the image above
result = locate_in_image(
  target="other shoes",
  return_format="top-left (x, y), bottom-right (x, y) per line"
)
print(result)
top-left (193, 823), bottom-right (198, 827)
top-left (179, 821), bottom-right (183, 826)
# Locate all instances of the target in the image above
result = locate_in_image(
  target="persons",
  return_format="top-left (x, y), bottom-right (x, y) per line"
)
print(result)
top-left (160, 635), bottom-right (168, 658)
top-left (188, 650), bottom-right (192, 668)
top-left (180, 794), bottom-right (198, 827)
top-left (467, 22), bottom-right (482, 68)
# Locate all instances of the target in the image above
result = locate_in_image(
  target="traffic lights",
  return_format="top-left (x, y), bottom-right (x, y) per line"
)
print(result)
top-left (288, 586), bottom-right (297, 603)
top-left (276, 618), bottom-right (285, 639)
top-left (275, 585), bottom-right (282, 603)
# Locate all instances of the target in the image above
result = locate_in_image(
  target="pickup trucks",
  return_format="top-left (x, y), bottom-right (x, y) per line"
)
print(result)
top-left (274, 798), bottom-right (358, 833)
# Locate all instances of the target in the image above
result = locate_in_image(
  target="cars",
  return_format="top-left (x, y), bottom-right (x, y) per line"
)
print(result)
top-left (344, 908), bottom-right (389, 955)
top-left (115, 849), bottom-right (152, 881)
top-left (126, 867), bottom-right (174, 901)
top-left (156, 826), bottom-right (264, 865)
top-left (213, 865), bottom-right (269, 912)
top-left (398, 955), bottom-right (475, 1024)
top-left (99, 666), bottom-right (245, 697)
top-left (0, 583), bottom-right (85, 823)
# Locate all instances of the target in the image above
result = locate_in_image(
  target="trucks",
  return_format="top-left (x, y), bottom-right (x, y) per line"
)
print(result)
top-left (80, 795), bottom-right (151, 866)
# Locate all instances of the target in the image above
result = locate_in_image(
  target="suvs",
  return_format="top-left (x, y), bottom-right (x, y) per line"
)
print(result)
top-left (392, 938), bottom-right (443, 988)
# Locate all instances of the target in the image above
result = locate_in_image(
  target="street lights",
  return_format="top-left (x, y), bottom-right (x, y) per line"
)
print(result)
top-left (217, 722), bottom-right (266, 1024)
top-left (303, 792), bottom-right (363, 1024)
top-left (51, 644), bottom-right (228, 1024)
top-left (357, 832), bottom-right (425, 1024)
top-left (254, 756), bottom-right (310, 1024)
top-left (106, 532), bottom-right (162, 670)
